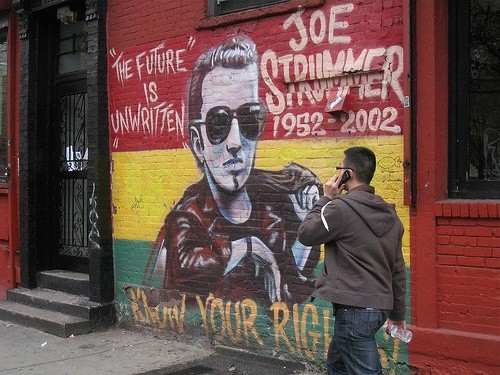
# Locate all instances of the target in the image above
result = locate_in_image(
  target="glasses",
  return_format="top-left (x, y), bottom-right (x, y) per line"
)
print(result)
top-left (335, 166), bottom-right (353, 173)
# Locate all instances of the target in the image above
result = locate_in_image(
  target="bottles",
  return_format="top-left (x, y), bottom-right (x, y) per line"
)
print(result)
top-left (382, 324), bottom-right (413, 342)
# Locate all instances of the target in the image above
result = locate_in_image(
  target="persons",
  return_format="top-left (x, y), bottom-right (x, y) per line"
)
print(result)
top-left (297, 147), bottom-right (407, 375)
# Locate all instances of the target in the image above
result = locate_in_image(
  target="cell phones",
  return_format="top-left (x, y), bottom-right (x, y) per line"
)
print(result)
top-left (335, 170), bottom-right (351, 188)
